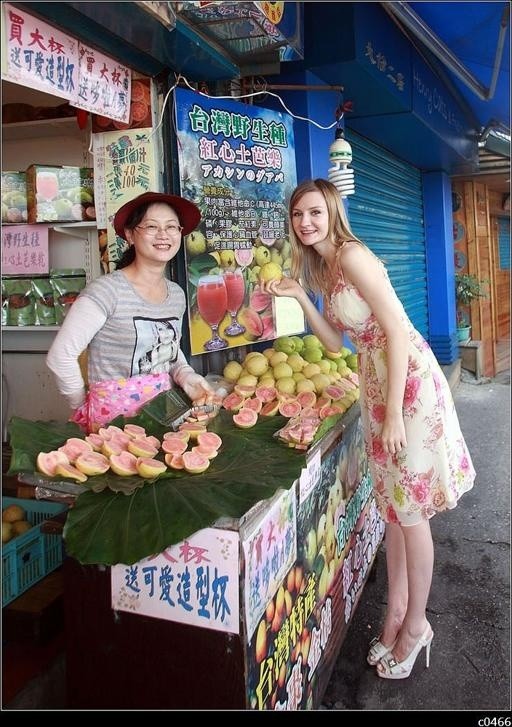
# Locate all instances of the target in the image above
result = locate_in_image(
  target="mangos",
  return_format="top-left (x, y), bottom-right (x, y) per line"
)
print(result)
top-left (3, 505), bottom-right (32, 545)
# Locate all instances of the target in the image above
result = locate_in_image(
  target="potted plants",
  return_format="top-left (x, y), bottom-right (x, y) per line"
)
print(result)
top-left (455, 273), bottom-right (492, 341)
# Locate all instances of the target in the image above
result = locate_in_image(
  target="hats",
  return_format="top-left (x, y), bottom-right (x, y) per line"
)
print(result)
top-left (114, 192), bottom-right (200, 240)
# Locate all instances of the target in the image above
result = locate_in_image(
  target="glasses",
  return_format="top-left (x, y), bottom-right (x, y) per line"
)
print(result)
top-left (134, 223), bottom-right (184, 237)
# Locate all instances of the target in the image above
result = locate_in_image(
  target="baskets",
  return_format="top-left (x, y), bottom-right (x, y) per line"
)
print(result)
top-left (2, 496), bottom-right (68, 610)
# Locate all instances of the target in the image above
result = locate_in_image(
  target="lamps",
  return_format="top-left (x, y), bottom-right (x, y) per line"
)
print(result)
top-left (241, 81), bottom-right (357, 201)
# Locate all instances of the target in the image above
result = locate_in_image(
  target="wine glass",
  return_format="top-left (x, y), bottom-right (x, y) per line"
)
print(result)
top-left (196, 270), bottom-right (246, 352)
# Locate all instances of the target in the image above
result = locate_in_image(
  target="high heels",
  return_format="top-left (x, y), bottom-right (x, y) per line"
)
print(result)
top-left (367, 617), bottom-right (435, 679)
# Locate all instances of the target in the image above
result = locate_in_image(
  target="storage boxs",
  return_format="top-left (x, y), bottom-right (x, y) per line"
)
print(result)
top-left (1, 164), bottom-right (96, 225)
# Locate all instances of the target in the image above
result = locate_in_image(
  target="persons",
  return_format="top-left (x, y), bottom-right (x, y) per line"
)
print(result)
top-left (44, 190), bottom-right (216, 438)
top-left (257, 177), bottom-right (476, 681)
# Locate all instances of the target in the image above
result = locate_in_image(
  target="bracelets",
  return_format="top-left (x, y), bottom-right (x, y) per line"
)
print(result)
top-left (297, 290), bottom-right (307, 301)
